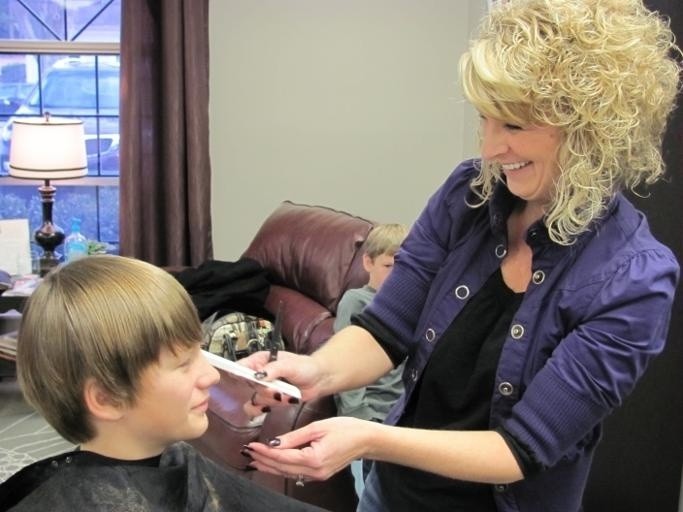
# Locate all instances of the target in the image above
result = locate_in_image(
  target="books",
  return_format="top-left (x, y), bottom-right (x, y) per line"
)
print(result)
top-left (0, 270), bottom-right (46, 359)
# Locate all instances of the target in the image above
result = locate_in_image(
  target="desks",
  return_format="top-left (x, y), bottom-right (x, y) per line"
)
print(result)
top-left (0, 296), bottom-right (28, 372)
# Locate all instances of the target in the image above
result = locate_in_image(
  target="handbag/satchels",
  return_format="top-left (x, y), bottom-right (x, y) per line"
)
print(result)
top-left (201, 312), bottom-right (286, 362)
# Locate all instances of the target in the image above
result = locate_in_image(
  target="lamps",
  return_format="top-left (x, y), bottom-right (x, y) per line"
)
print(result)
top-left (9, 112), bottom-right (89, 279)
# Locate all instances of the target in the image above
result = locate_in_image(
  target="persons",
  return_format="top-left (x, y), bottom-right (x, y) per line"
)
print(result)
top-left (235, 0), bottom-right (683, 512)
top-left (334, 224), bottom-right (410, 498)
top-left (10, 253), bottom-right (327, 511)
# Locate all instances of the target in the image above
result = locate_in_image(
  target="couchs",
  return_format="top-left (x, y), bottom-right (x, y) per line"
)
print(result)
top-left (183, 201), bottom-right (376, 512)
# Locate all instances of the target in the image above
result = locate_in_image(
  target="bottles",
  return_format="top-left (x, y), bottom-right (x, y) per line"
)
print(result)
top-left (64, 219), bottom-right (88, 263)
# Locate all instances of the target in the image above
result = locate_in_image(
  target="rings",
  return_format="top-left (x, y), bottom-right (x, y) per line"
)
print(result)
top-left (295, 473), bottom-right (307, 488)
